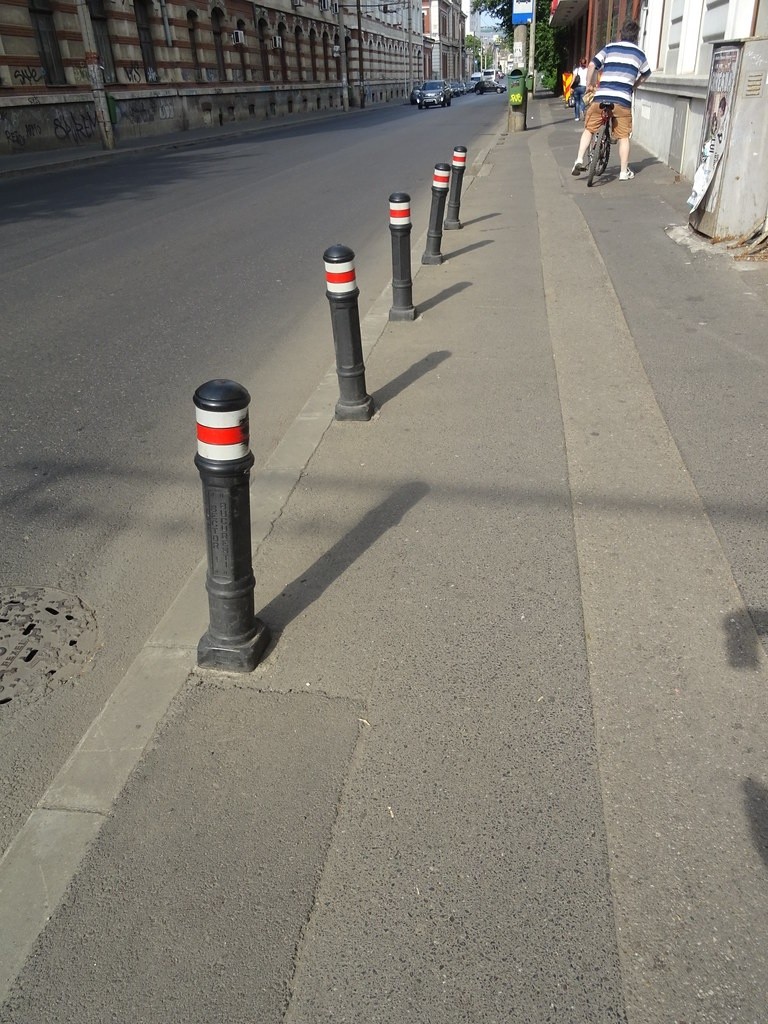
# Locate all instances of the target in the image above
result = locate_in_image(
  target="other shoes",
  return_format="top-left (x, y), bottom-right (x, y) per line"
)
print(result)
top-left (575, 118), bottom-right (580, 121)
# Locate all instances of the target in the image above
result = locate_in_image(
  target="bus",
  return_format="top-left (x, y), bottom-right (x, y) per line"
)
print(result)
top-left (482, 69), bottom-right (500, 84)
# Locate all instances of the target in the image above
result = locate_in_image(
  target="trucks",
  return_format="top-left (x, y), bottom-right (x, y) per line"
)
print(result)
top-left (471, 72), bottom-right (483, 82)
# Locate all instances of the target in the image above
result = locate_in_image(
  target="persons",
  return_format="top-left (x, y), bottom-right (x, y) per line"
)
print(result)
top-left (571, 21), bottom-right (652, 180)
top-left (568, 58), bottom-right (588, 121)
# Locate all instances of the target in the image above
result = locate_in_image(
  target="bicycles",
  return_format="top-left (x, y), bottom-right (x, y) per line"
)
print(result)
top-left (577, 101), bottom-right (615, 187)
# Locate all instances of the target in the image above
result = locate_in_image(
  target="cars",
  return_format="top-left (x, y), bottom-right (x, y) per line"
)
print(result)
top-left (417, 80), bottom-right (452, 110)
top-left (448, 81), bottom-right (478, 99)
top-left (474, 80), bottom-right (507, 95)
top-left (410, 86), bottom-right (423, 105)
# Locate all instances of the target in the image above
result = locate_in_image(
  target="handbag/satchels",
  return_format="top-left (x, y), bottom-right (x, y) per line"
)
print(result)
top-left (571, 75), bottom-right (580, 89)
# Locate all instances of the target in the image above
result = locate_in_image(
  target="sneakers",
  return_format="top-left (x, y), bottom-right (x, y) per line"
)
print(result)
top-left (572, 159), bottom-right (582, 176)
top-left (619, 168), bottom-right (635, 181)
